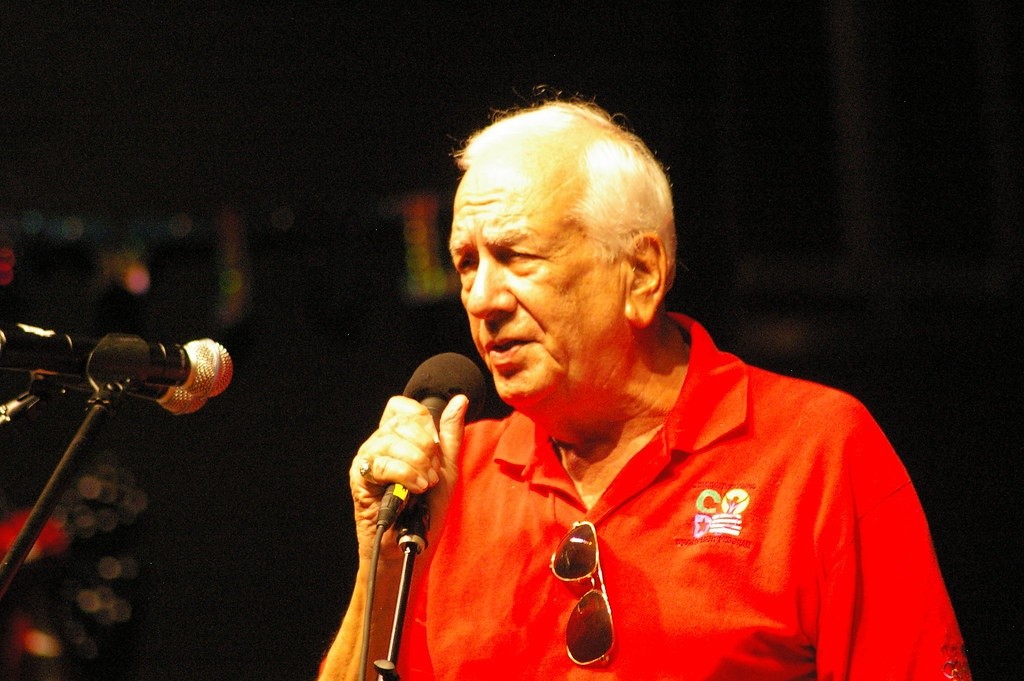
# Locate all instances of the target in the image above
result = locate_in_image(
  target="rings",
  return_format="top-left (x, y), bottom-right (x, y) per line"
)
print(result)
top-left (359, 455), bottom-right (384, 485)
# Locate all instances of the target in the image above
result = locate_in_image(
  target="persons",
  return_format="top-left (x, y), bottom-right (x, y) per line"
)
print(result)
top-left (317, 102), bottom-right (977, 681)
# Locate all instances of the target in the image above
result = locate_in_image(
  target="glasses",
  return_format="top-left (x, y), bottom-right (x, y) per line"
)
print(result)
top-left (545, 519), bottom-right (617, 670)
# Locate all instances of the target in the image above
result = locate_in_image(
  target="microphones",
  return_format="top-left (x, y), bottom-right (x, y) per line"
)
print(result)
top-left (2, 352), bottom-right (204, 415)
top-left (2, 306), bottom-right (233, 398)
top-left (373, 350), bottom-right (487, 527)
